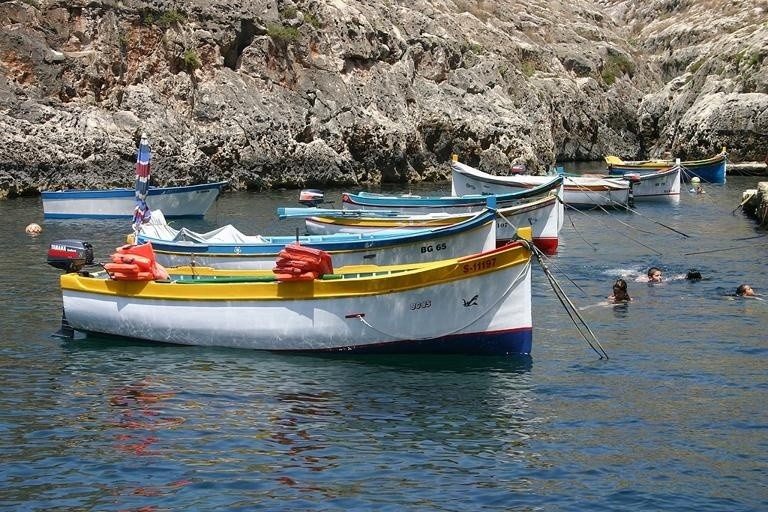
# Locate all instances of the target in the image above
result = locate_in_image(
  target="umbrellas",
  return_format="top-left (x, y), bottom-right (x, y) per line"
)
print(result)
top-left (131, 130), bottom-right (152, 243)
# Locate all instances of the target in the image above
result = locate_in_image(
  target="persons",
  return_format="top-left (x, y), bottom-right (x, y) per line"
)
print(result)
top-left (689, 177), bottom-right (705, 193)
top-left (687, 269), bottom-right (702, 279)
top-left (648, 266), bottom-right (663, 281)
top-left (736, 283), bottom-right (756, 297)
top-left (607, 277), bottom-right (633, 302)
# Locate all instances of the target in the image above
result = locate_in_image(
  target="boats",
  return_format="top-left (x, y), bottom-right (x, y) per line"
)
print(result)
top-left (124, 195), bottom-right (497, 271)
top-left (58, 240), bottom-right (533, 352)
top-left (40, 179), bottom-right (227, 221)
